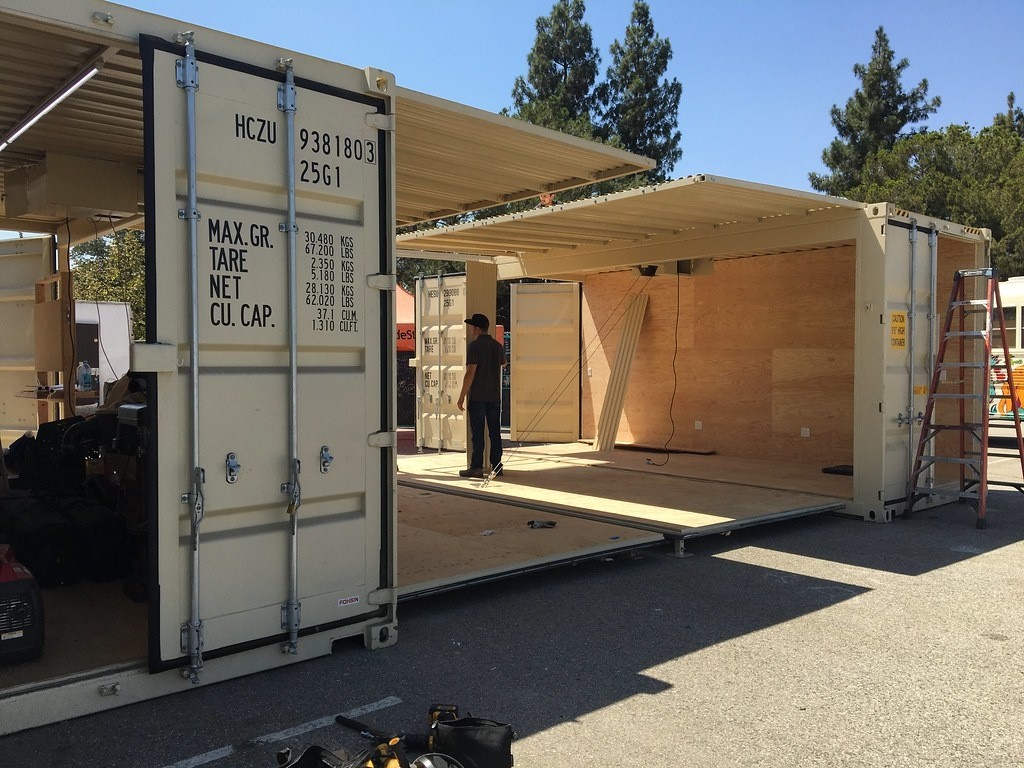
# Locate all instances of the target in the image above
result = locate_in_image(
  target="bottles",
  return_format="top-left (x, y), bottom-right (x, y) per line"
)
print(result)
top-left (76, 360), bottom-right (91, 391)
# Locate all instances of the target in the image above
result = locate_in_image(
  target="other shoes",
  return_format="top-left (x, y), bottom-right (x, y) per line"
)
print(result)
top-left (495, 470), bottom-right (503, 475)
top-left (459, 468), bottom-right (483, 477)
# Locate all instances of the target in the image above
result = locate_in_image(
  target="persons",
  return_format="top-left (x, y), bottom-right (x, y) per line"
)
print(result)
top-left (456, 313), bottom-right (504, 477)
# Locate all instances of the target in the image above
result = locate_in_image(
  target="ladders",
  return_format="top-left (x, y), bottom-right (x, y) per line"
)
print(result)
top-left (902, 268), bottom-right (1023, 533)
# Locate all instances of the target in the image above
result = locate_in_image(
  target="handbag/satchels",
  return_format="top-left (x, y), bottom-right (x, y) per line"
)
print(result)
top-left (433, 717), bottom-right (516, 768)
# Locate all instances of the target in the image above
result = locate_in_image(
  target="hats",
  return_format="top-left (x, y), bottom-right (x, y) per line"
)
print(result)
top-left (464, 313), bottom-right (489, 328)
